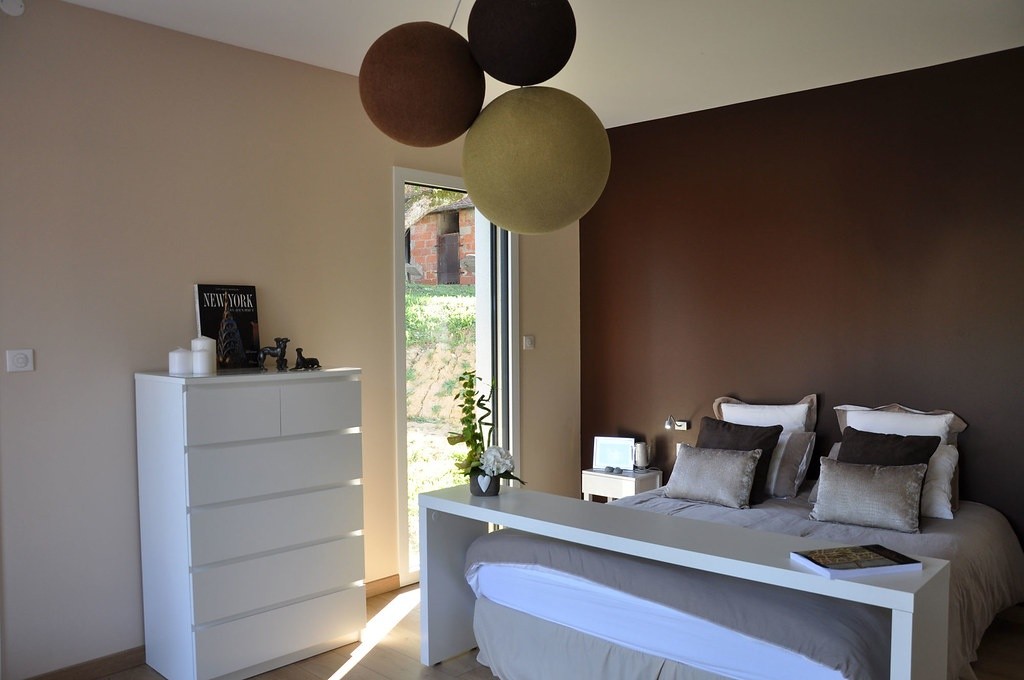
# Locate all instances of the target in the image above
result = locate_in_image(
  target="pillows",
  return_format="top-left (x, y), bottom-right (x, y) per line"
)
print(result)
top-left (763, 429), bottom-right (818, 500)
top-left (830, 426), bottom-right (940, 532)
top-left (694, 416), bottom-right (783, 503)
top-left (713, 393), bottom-right (820, 433)
top-left (832, 405), bottom-right (970, 445)
top-left (666, 444), bottom-right (758, 511)
top-left (814, 456), bottom-right (934, 533)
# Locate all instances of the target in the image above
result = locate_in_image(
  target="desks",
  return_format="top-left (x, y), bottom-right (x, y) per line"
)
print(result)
top-left (417, 485), bottom-right (950, 679)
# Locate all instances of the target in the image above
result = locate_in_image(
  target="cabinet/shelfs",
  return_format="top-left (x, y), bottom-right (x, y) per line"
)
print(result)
top-left (134, 369), bottom-right (369, 680)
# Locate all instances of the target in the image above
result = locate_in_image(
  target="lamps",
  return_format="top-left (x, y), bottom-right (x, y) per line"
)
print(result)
top-left (665, 415), bottom-right (691, 432)
top-left (356, 0), bottom-right (613, 236)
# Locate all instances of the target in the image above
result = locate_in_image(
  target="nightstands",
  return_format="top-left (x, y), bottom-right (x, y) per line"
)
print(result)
top-left (580, 467), bottom-right (663, 504)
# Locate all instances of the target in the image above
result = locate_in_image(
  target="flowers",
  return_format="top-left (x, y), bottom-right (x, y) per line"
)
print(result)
top-left (445, 371), bottom-right (526, 485)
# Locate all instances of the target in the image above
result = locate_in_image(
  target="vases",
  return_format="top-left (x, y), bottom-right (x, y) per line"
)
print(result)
top-left (468, 468), bottom-right (502, 495)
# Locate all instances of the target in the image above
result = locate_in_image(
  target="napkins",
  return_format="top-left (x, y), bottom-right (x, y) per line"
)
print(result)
top-left (828, 441), bottom-right (960, 519)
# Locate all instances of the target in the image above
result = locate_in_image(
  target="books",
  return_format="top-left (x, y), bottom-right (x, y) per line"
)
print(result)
top-left (790, 544), bottom-right (922, 579)
top-left (194, 284), bottom-right (261, 369)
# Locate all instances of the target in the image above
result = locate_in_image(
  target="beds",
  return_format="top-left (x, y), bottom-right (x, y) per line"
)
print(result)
top-left (458, 486), bottom-right (1023, 680)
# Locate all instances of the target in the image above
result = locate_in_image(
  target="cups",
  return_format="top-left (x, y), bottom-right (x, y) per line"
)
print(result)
top-left (168, 339), bottom-right (216, 378)
